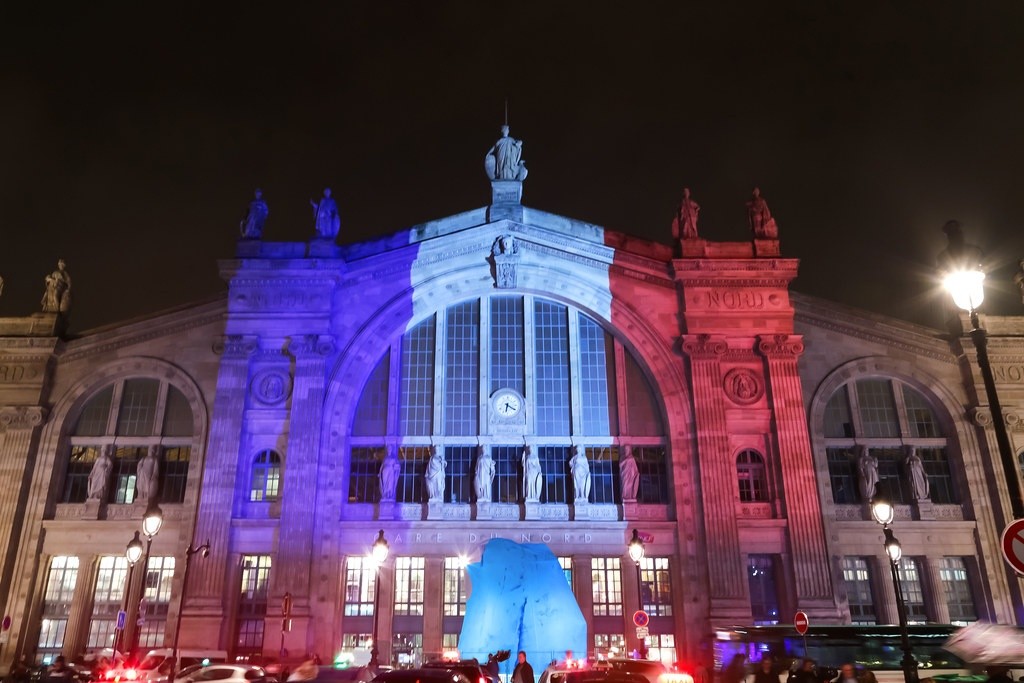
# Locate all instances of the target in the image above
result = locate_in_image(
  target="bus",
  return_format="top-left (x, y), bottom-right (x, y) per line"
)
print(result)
top-left (711, 620), bottom-right (1024, 683)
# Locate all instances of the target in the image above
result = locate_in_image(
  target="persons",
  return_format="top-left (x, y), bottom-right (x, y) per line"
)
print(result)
top-left (720, 652), bottom-right (880, 683)
top-left (745, 186), bottom-right (771, 240)
top-left (618, 445), bottom-right (640, 499)
top-left (486, 125), bottom-right (521, 179)
top-left (376, 445), bottom-right (402, 502)
top-left (568, 445), bottom-right (589, 499)
top-left (137, 445), bottom-right (159, 502)
top-left (903, 445), bottom-right (930, 499)
top-left (511, 650), bottom-right (536, 683)
top-left (86, 445), bottom-right (113, 500)
top-left (474, 444), bottom-right (496, 503)
top-left (240, 188), bottom-right (269, 237)
top-left (856, 446), bottom-right (880, 504)
top-left (424, 444), bottom-right (448, 503)
top-left (40, 258), bottom-right (72, 310)
top-left (309, 188), bottom-right (340, 240)
top-left (674, 187), bottom-right (700, 239)
top-left (521, 446), bottom-right (542, 504)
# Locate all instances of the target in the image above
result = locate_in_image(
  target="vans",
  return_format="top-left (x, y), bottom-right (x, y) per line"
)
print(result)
top-left (127, 648), bottom-right (230, 683)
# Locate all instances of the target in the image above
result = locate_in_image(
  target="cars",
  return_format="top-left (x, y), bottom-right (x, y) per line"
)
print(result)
top-left (10, 662), bottom-right (93, 683)
top-left (147, 662), bottom-right (278, 683)
top-left (285, 663), bottom-right (503, 683)
top-left (536, 659), bottom-right (694, 683)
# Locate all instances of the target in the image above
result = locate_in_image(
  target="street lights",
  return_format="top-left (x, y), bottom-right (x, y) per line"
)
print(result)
top-left (127, 496), bottom-right (164, 653)
top-left (933, 215), bottom-right (1024, 520)
top-left (111, 530), bottom-right (143, 669)
top-left (369, 528), bottom-right (390, 668)
top-left (627, 528), bottom-right (646, 660)
top-left (884, 529), bottom-right (908, 624)
top-left (870, 480), bottom-right (919, 683)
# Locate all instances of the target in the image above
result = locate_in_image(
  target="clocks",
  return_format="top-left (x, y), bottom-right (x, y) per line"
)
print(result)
top-left (498, 394), bottom-right (520, 416)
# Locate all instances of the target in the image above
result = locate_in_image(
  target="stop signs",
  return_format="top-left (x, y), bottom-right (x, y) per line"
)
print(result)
top-left (794, 612), bottom-right (809, 635)
